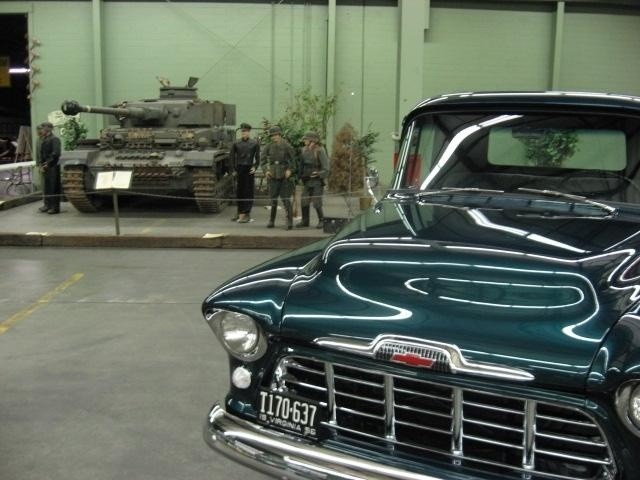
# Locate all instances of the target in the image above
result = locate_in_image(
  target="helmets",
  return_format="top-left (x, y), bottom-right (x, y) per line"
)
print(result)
top-left (268, 125), bottom-right (282, 137)
top-left (302, 131), bottom-right (315, 142)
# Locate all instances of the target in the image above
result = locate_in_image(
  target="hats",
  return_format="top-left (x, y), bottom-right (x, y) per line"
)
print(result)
top-left (240, 123), bottom-right (252, 132)
top-left (36, 123), bottom-right (53, 130)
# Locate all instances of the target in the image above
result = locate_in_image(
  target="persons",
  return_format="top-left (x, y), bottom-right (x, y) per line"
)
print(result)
top-left (230, 123), bottom-right (260, 222)
top-left (296, 132), bottom-right (330, 229)
top-left (40, 121), bottom-right (61, 213)
top-left (261, 126), bottom-right (296, 229)
top-left (35, 124), bottom-right (46, 205)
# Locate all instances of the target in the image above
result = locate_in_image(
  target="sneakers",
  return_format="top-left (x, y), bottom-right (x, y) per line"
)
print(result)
top-left (38, 204), bottom-right (60, 214)
top-left (231, 213), bottom-right (250, 224)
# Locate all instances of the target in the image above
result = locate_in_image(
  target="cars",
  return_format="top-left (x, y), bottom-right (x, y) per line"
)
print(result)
top-left (201, 87), bottom-right (638, 480)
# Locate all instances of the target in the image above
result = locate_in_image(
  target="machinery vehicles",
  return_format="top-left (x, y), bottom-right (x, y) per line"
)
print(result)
top-left (56, 74), bottom-right (249, 214)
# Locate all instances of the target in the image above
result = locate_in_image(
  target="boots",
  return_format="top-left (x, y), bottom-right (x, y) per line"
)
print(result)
top-left (296, 206), bottom-right (310, 228)
top-left (288, 212), bottom-right (293, 230)
top-left (316, 208), bottom-right (326, 229)
top-left (266, 205), bottom-right (277, 228)
